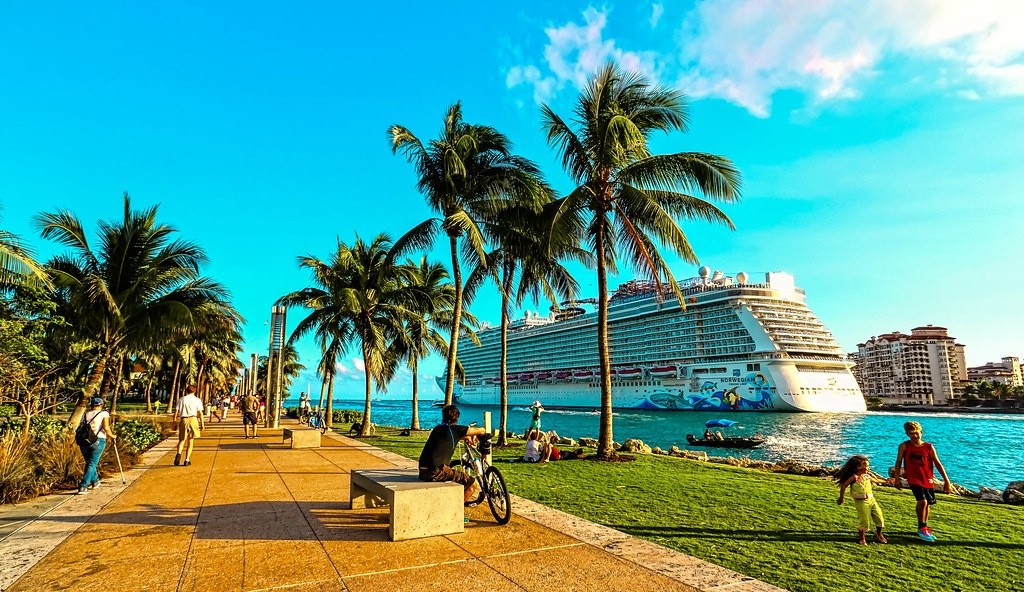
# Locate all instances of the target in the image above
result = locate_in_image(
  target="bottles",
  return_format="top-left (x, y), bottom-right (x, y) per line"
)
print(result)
top-left (465, 466), bottom-right (470, 476)
top-left (474, 457), bottom-right (482, 475)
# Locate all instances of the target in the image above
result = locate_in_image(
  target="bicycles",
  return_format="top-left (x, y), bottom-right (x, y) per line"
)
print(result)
top-left (449, 421), bottom-right (512, 525)
top-left (308, 411), bottom-right (327, 435)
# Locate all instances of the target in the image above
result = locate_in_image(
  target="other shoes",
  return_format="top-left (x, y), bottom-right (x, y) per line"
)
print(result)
top-left (464, 517), bottom-right (470, 523)
top-left (874, 532), bottom-right (888, 544)
top-left (860, 540), bottom-right (868, 546)
top-left (253, 435), bottom-right (260, 438)
top-left (174, 454), bottom-right (182, 466)
top-left (184, 460), bottom-right (191, 466)
top-left (917, 527), bottom-right (936, 543)
top-left (246, 436), bottom-right (250, 439)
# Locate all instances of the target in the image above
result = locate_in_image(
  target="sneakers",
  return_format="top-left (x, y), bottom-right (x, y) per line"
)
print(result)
top-left (78, 487), bottom-right (89, 494)
top-left (92, 481), bottom-right (101, 489)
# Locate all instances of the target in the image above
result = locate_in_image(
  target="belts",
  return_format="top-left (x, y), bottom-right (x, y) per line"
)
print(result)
top-left (181, 416), bottom-right (197, 419)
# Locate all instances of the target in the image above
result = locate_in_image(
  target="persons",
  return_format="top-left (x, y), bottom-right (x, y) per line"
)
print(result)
top-left (206, 388), bottom-right (265, 439)
top-left (76, 398), bottom-right (116, 494)
top-left (894, 421), bottom-right (954, 543)
top-left (522, 400), bottom-right (544, 441)
top-left (299, 391), bottom-right (309, 425)
top-left (704, 429), bottom-right (715, 442)
top-left (833, 455), bottom-right (895, 546)
top-left (543, 435), bottom-right (587, 463)
top-left (519, 433), bottom-right (544, 463)
top-left (173, 385), bottom-right (204, 466)
top-left (154, 399), bottom-right (162, 416)
top-left (418, 405), bottom-right (485, 523)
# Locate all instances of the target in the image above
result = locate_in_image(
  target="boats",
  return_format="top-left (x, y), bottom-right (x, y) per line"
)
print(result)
top-left (519, 374), bottom-right (534, 381)
top-left (535, 373), bottom-right (552, 380)
top-left (686, 420), bottom-right (764, 448)
top-left (650, 366), bottom-right (676, 378)
top-left (594, 370), bottom-right (617, 378)
top-left (618, 368), bottom-right (641, 377)
top-left (492, 375), bottom-right (518, 384)
top-left (552, 372), bottom-right (572, 380)
top-left (573, 370), bottom-right (593, 379)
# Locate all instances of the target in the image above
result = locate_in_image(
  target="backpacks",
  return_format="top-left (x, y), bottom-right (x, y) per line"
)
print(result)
top-left (75, 411), bottom-right (104, 447)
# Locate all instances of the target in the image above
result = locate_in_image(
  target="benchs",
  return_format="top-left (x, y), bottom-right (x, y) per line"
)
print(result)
top-left (283, 427), bottom-right (321, 449)
top-left (350, 469), bottom-right (464, 541)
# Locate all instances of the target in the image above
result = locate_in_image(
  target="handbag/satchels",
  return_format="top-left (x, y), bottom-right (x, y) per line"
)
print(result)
top-left (224, 402), bottom-right (229, 407)
top-left (532, 407), bottom-right (540, 420)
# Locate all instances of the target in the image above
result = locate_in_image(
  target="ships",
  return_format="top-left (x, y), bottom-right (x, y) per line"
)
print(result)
top-left (434, 265), bottom-right (868, 413)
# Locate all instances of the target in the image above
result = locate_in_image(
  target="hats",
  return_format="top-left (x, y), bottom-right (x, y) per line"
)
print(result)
top-left (533, 401), bottom-right (541, 407)
top-left (91, 398), bottom-right (104, 406)
